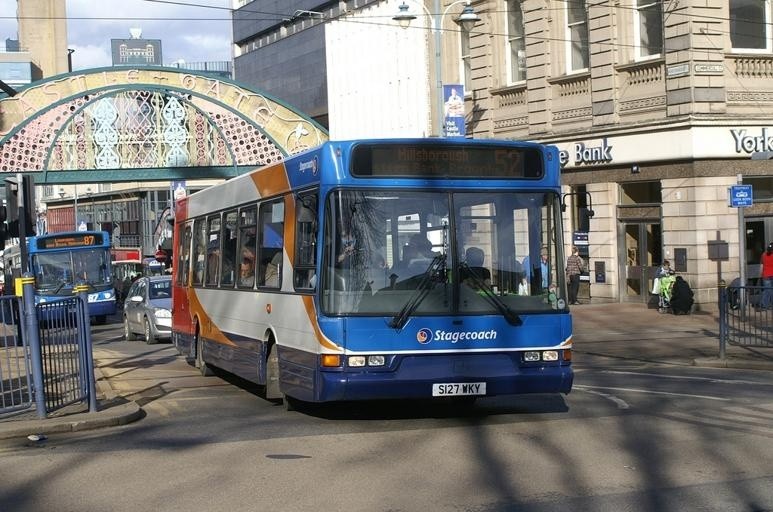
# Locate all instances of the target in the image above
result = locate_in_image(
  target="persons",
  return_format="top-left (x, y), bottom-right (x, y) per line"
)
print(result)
top-left (448, 88), bottom-right (463, 107)
top-left (334, 226), bottom-right (491, 281)
top-left (565, 247), bottom-right (584, 305)
top-left (656, 260), bottom-right (694, 315)
top-left (206, 226), bottom-right (236, 287)
top-left (521, 255), bottom-right (531, 296)
top-left (540, 249), bottom-right (549, 289)
top-left (760, 242), bottom-right (773, 310)
top-left (241, 226), bottom-right (256, 286)
top-left (516, 260), bottom-right (522, 294)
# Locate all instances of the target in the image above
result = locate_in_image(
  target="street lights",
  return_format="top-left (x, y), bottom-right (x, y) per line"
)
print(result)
top-left (391, 0), bottom-right (481, 137)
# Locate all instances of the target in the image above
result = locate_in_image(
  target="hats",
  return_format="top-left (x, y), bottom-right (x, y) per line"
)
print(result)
top-left (540, 249), bottom-right (548, 255)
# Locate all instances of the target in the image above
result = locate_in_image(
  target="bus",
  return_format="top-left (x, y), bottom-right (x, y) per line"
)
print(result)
top-left (1, 230), bottom-right (119, 327)
top-left (169, 137), bottom-right (576, 405)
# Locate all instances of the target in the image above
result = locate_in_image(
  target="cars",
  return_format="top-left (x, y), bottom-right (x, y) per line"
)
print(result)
top-left (122, 271), bottom-right (173, 344)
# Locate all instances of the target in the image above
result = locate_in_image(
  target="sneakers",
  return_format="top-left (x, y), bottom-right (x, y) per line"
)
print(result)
top-left (677, 309), bottom-right (691, 315)
top-left (569, 300), bottom-right (583, 305)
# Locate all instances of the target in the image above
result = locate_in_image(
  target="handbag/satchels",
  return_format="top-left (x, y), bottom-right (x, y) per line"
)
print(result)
top-left (652, 278), bottom-right (661, 294)
